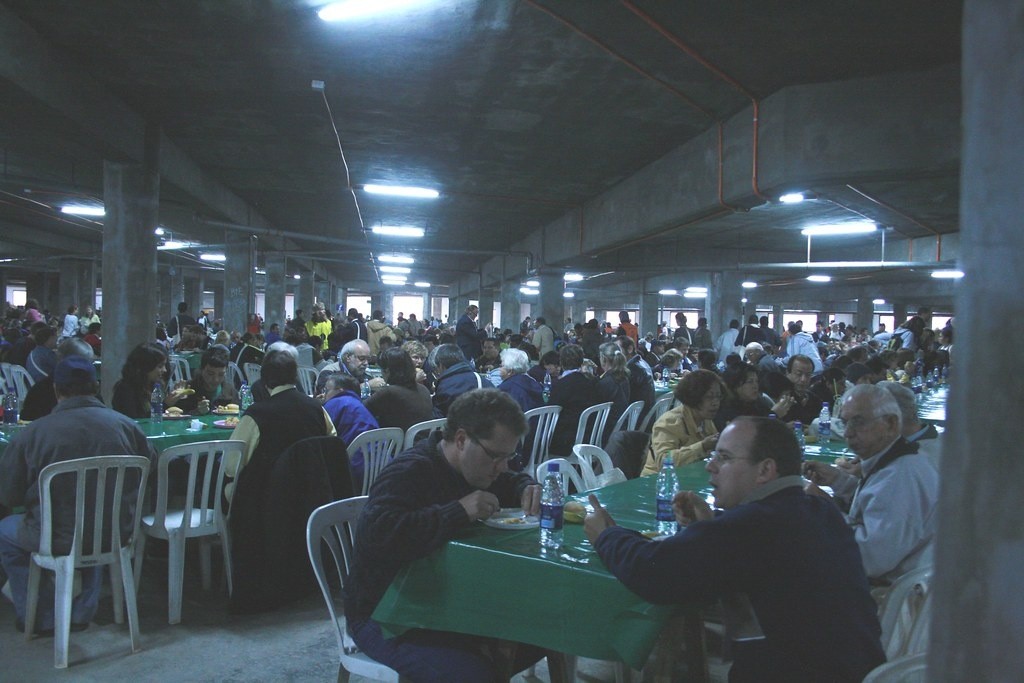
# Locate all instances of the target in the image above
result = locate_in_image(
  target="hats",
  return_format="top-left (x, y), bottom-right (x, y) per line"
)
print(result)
top-left (847, 362), bottom-right (873, 379)
top-left (312, 302), bottom-right (326, 311)
top-left (54, 355), bottom-right (97, 385)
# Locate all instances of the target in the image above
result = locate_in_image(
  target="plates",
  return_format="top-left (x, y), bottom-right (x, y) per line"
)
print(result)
top-left (162, 413), bottom-right (191, 419)
top-left (211, 410), bottom-right (238, 415)
top-left (481, 507), bottom-right (540, 529)
top-left (180, 350), bottom-right (194, 354)
top-left (213, 420), bottom-right (236, 428)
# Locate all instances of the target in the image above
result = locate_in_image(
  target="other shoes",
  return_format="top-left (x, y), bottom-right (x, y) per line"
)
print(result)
top-left (15, 617), bottom-right (89, 637)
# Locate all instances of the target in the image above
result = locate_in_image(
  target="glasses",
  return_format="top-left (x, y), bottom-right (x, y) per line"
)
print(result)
top-left (835, 414), bottom-right (888, 433)
top-left (704, 392), bottom-right (725, 401)
top-left (468, 432), bottom-right (518, 464)
top-left (708, 453), bottom-right (754, 467)
top-left (472, 312), bottom-right (477, 316)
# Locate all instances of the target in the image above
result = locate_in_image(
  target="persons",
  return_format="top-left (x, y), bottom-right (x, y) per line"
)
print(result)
top-left (0, 298), bottom-right (954, 637)
top-left (583, 416), bottom-right (888, 683)
top-left (344, 388), bottom-right (545, 683)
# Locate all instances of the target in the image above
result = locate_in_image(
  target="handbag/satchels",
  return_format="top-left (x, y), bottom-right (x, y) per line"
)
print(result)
top-left (735, 345), bottom-right (745, 361)
top-left (173, 334), bottom-right (181, 345)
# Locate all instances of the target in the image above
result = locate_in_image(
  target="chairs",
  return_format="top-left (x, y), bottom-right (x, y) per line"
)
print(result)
top-left (403, 417), bottom-right (448, 451)
top-left (305, 493), bottom-right (570, 683)
top-left (656, 391), bottom-right (675, 419)
top-left (639, 397), bottom-right (672, 431)
top-left (225, 361), bottom-right (244, 387)
top-left (132, 439), bottom-right (248, 625)
top-left (595, 431), bottom-right (650, 480)
top-left (0, 356), bottom-right (193, 407)
top-left (346, 427), bottom-right (405, 495)
top-left (548, 400), bottom-right (615, 470)
top-left (232, 435), bottom-right (356, 614)
top-left (573, 444), bottom-right (627, 489)
top-left (535, 457), bottom-right (588, 497)
top-left (23, 454), bottom-right (151, 669)
top-left (244, 362), bottom-right (262, 385)
top-left (296, 363), bottom-right (320, 395)
top-left (879, 559), bottom-right (933, 660)
top-left (614, 400), bottom-right (645, 431)
top-left (506, 404), bottom-right (562, 481)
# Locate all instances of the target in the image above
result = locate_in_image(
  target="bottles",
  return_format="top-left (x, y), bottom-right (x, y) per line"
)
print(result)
top-left (150, 384), bottom-right (163, 423)
top-left (361, 379), bottom-right (371, 399)
top-left (470, 359), bottom-right (475, 367)
top-left (538, 463), bottom-right (565, 549)
top-left (238, 380), bottom-right (253, 417)
top-left (655, 458), bottom-right (680, 534)
top-left (911, 364), bottom-right (946, 404)
top-left (662, 368), bottom-right (668, 386)
top-left (793, 422), bottom-right (806, 462)
top-left (818, 402), bottom-right (831, 444)
top-left (485, 372), bottom-right (492, 382)
top-left (543, 371), bottom-right (551, 392)
top-left (3, 387), bottom-right (20, 439)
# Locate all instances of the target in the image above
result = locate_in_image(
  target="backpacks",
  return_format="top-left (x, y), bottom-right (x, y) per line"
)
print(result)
top-left (889, 335), bottom-right (902, 351)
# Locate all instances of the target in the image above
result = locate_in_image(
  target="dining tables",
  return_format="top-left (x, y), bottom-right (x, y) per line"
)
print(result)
top-left (369, 439), bottom-right (857, 683)
top-left (654, 379), bottom-right (679, 406)
top-left (169, 351), bottom-right (202, 380)
top-left (0, 407), bottom-right (245, 516)
top-left (911, 374), bottom-right (949, 428)
